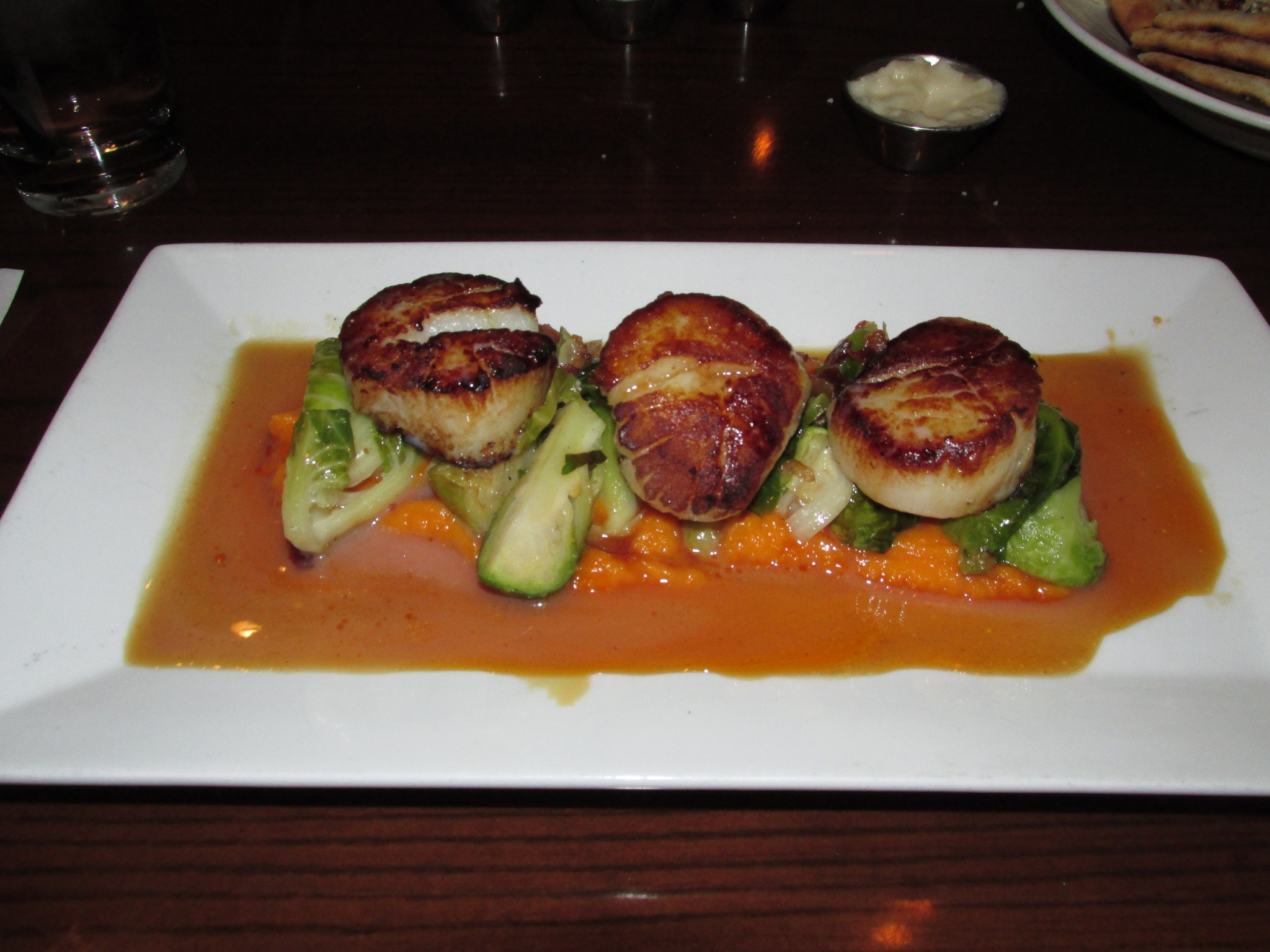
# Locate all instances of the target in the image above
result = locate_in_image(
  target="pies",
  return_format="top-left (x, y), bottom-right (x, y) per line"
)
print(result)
top-left (1108, 0), bottom-right (1270, 113)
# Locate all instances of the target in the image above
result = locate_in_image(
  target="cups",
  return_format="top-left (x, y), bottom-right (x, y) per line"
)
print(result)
top-left (599, 0), bottom-right (655, 40)
top-left (1, 1), bottom-right (191, 218)
top-left (467, 0), bottom-right (518, 34)
top-left (727, 0), bottom-right (772, 22)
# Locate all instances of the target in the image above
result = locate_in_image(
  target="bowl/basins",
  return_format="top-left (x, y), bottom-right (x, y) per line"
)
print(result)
top-left (848, 56), bottom-right (1013, 174)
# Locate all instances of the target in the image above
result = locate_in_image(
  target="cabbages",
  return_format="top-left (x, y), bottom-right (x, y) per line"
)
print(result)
top-left (267, 328), bottom-right (1082, 575)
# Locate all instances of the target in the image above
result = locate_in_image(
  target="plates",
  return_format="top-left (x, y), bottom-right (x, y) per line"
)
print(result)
top-left (1, 241), bottom-right (1270, 798)
top-left (1042, 0), bottom-right (1270, 132)
